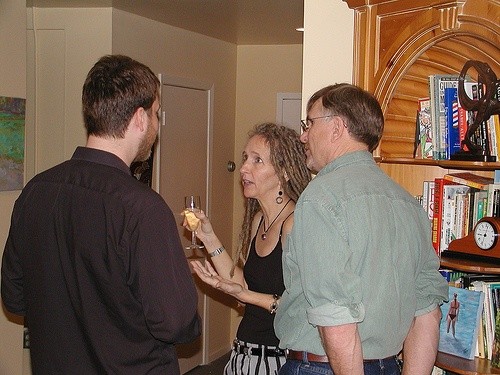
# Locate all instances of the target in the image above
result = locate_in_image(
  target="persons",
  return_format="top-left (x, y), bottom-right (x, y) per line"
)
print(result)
top-left (1, 55), bottom-right (197, 375)
top-left (446, 291), bottom-right (460, 336)
top-left (272, 82), bottom-right (439, 373)
top-left (178, 122), bottom-right (313, 373)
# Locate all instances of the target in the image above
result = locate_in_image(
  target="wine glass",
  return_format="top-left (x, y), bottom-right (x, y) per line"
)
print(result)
top-left (184, 195), bottom-right (204, 250)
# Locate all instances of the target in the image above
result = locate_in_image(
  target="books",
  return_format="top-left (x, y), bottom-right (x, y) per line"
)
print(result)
top-left (417, 74), bottom-right (499, 160)
top-left (436, 269), bottom-right (499, 361)
top-left (416, 169), bottom-right (500, 255)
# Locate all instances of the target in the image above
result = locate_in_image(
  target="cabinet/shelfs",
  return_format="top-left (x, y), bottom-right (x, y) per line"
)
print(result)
top-left (371, 156), bottom-right (500, 375)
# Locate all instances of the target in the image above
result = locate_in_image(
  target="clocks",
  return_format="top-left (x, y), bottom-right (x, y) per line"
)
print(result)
top-left (473, 217), bottom-right (500, 251)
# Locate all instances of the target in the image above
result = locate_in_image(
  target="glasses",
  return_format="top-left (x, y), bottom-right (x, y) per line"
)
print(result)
top-left (300, 115), bottom-right (348, 132)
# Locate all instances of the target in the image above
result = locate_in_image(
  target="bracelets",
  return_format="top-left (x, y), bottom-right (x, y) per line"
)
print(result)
top-left (270, 293), bottom-right (279, 313)
top-left (205, 245), bottom-right (227, 258)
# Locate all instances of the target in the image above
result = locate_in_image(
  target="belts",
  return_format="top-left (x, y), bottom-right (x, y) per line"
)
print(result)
top-left (232, 340), bottom-right (285, 357)
top-left (288, 350), bottom-right (330, 363)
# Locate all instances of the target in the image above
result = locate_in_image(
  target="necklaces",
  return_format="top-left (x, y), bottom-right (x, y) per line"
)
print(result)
top-left (261, 198), bottom-right (290, 239)
